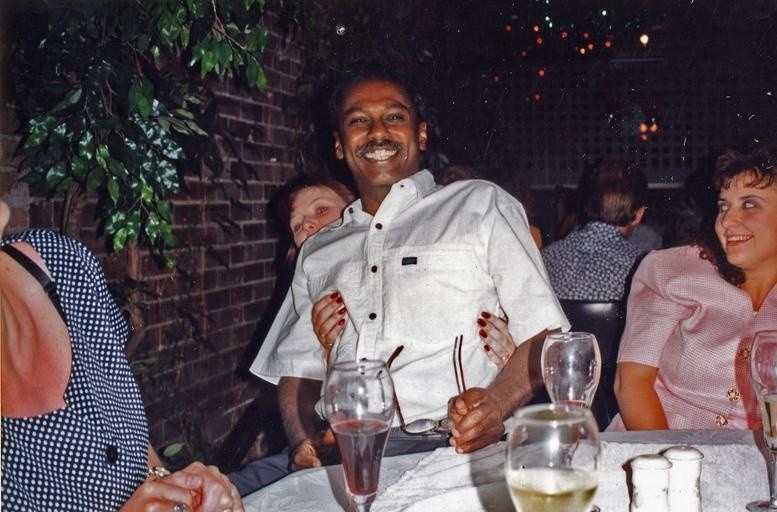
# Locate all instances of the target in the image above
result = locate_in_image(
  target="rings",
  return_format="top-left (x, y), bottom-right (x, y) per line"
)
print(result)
top-left (325, 342), bottom-right (333, 347)
top-left (319, 333), bottom-right (325, 337)
top-left (176, 504), bottom-right (186, 511)
top-left (502, 352), bottom-right (508, 360)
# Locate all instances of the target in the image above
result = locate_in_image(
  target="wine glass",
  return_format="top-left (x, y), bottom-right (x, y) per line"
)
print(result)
top-left (322, 361), bottom-right (393, 512)
top-left (746, 330), bottom-right (777, 512)
top-left (505, 404), bottom-right (600, 512)
top-left (541, 333), bottom-right (601, 413)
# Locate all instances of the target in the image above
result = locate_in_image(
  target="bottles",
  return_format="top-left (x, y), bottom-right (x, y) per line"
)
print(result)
top-left (629, 446), bottom-right (704, 511)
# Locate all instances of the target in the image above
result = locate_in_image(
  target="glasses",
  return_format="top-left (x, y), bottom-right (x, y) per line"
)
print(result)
top-left (387, 335), bottom-right (466, 434)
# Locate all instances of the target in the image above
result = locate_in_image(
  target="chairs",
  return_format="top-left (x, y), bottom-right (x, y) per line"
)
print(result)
top-left (561, 298), bottom-right (628, 430)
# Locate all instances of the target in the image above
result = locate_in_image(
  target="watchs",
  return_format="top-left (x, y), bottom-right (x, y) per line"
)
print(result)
top-left (146, 464), bottom-right (170, 478)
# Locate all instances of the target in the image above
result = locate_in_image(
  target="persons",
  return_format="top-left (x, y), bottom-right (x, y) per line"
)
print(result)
top-left (281, 176), bottom-right (517, 366)
top-left (601, 148), bottom-right (776, 431)
top-left (3, 190), bottom-right (246, 512)
top-left (224, 64), bottom-right (571, 497)
top-left (429, 147), bottom-right (722, 303)
